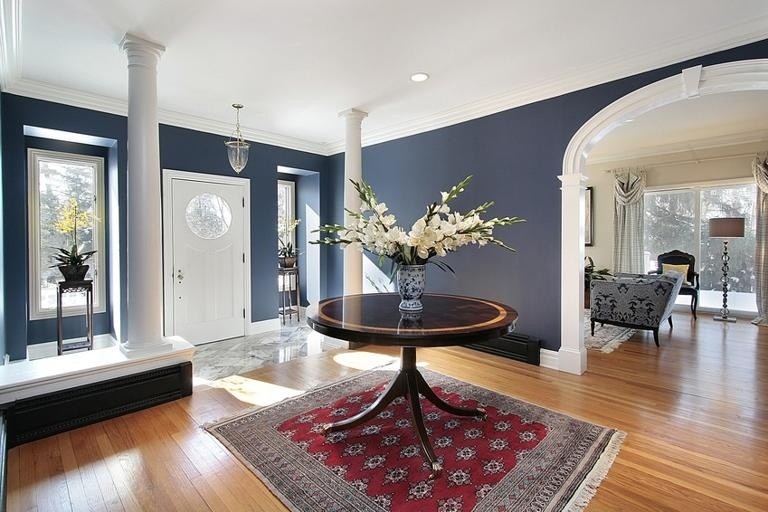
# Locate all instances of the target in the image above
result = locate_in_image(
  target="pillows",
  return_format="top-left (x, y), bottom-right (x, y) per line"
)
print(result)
top-left (661, 263), bottom-right (689, 285)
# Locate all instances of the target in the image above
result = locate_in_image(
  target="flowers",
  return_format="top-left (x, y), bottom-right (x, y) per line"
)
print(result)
top-left (309, 174), bottom-right (530, 285)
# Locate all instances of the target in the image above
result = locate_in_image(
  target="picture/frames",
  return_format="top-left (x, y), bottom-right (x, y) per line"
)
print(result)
top-left (585, 187), bottom-right (594, 247)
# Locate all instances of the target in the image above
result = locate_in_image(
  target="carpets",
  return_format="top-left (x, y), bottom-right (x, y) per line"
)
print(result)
top-left (199, 358), bottom-right (627, 512)
top-left (584, 307), bottom-right (635, 354)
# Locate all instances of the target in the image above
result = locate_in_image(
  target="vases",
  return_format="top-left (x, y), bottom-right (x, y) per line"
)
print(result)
top-left (396, 264), bottom-right (425, 313)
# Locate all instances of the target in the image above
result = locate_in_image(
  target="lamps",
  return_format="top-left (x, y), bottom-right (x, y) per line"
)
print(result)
top-left (224, 103), bottom-right (251, 175)
top-left (708, 217), bottom-right (745, 322)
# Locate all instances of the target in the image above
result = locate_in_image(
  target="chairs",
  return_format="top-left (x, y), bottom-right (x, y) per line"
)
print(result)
top-left (590, 269), bottom-right (686, 348)
top-left (648, 250), bottom-right (699, 320)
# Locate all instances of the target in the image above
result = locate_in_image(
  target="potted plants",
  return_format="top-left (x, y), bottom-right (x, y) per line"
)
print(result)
top-left (278, 216), bottom-right (301, 267)
top-left (48, 197), bottom-right (102, 281)
top-left (584, 256), bottom-right (613, 308)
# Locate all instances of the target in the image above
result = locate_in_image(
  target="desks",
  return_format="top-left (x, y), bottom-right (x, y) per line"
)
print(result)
top-left (280, 267), bottom-right (301, 325)
top-left (304, 292), bottom-right (518, 479)
top-left (55, 277), bottom-right (93, 355)
